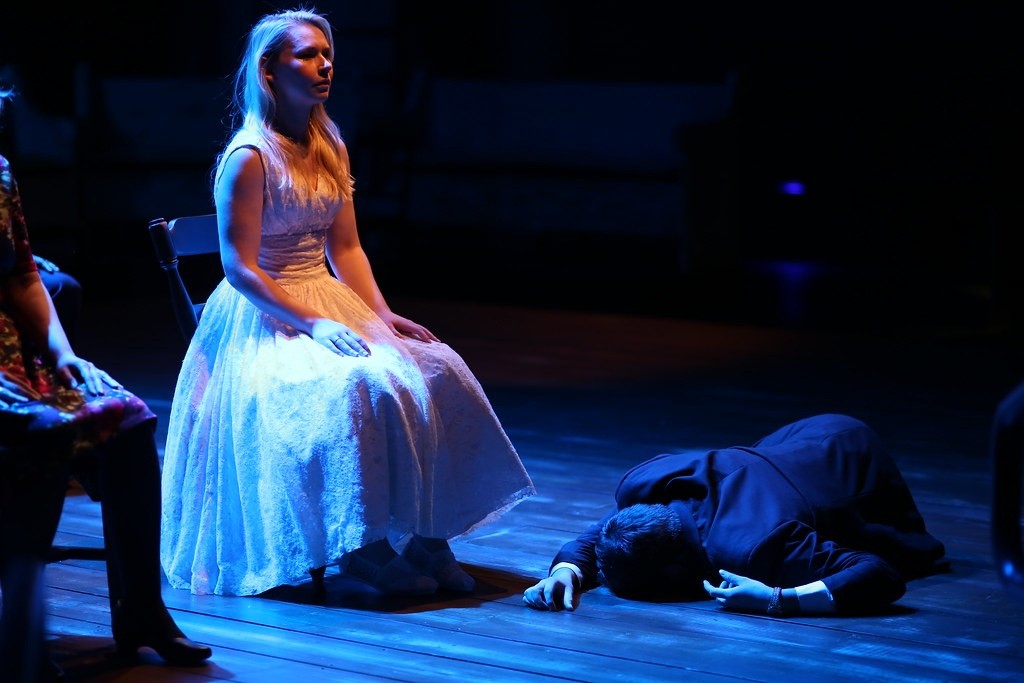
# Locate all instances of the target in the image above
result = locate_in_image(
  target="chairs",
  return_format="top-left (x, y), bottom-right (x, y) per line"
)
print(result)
top-left (148, 213), bottom-right (221, 345)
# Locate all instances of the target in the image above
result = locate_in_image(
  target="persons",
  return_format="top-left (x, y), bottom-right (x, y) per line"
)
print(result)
top-left (522, 413), bottom-right (949, 624)
top-left (163, 11), bottom-right (536, 609)
top-left (0, 90), bottom-right (215, 669)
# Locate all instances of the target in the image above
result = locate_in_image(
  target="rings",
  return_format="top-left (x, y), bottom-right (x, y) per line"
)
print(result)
top-left (334, 337), bottom-right (341, 345)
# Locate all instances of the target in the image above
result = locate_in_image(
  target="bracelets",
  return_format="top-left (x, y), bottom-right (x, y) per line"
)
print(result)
top-left (767, 586), bottom-right (783, 617)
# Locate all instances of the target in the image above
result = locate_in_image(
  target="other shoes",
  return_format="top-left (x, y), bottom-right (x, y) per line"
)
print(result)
top-left (339, 551), bottom-right (417, 586)
top-left (400, 538), bottom-right (479, 594)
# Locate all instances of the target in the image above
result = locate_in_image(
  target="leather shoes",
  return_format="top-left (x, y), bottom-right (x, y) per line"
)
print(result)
top-left (115, 622), bottom-right (213, 663)
